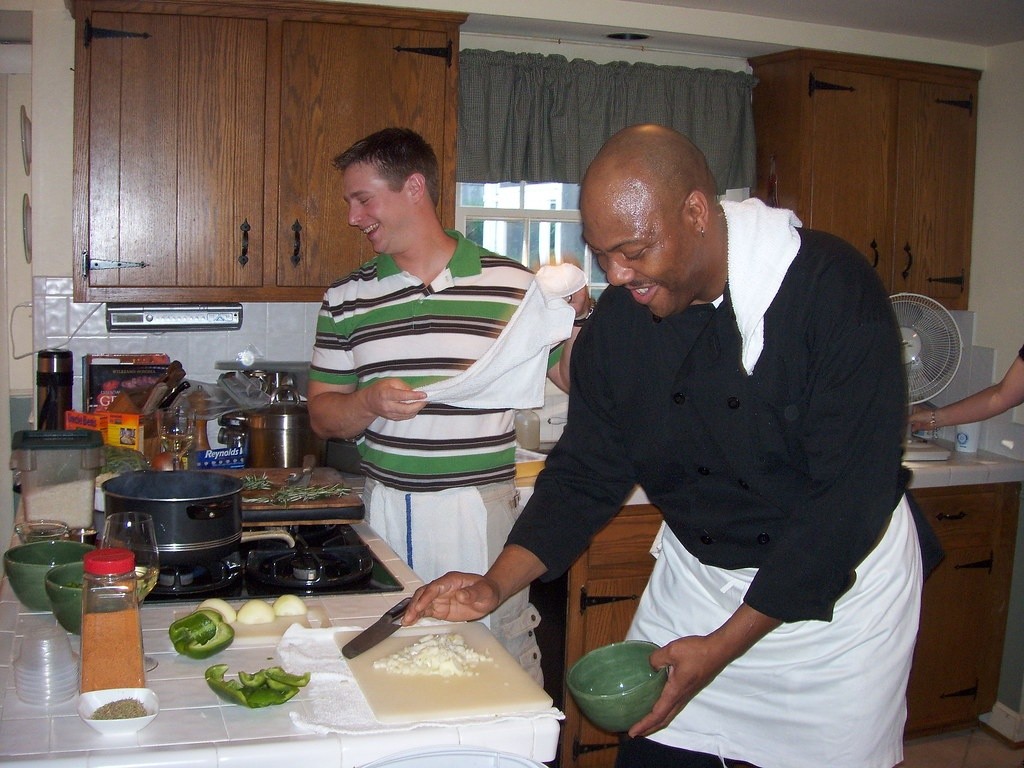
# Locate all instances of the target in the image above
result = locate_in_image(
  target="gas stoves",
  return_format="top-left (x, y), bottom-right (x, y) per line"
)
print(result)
top-left (144, 524), bottom-right (405, 604)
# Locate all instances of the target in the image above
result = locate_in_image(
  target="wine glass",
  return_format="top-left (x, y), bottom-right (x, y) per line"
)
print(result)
top-left (156, 407), bottom-right (195, 470)
top-left (100, 512), bottom-right (160, 672)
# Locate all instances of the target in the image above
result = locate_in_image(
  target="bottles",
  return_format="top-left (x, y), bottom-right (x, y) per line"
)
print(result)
top-left (188, 384), bottom-right (212, 452)
top-left (81, 548), bottom-right (146, 694)
top-left (514, 409), bottom-right (540, 450)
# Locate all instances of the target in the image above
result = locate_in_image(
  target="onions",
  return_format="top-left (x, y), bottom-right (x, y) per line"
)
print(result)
top-left (152, 451), bottom-right (186, 471)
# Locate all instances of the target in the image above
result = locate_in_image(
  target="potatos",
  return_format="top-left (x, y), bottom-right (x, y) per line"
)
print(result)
top-left (196, 594), bottom-right (307, 625)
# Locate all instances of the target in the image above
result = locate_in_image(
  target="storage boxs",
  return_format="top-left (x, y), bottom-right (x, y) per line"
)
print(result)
top-left (65, 409), bottom-right (144, 455)
top-left (82, 353), bottom-right (171, 412)
top-left (196, 447), bottom-right (246, 469)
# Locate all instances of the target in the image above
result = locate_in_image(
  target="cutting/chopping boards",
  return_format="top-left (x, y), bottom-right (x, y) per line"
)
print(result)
top-left (208, 468), bottom-right (363, 509)
top-left (334, 621), bottom-right (553, 724)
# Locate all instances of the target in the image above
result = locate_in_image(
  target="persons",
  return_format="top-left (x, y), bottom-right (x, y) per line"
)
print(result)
top-left (401, 125), bottom-right (942, 768)
top-left (909, 343), bottom-right (1024, 434)
top-left (307, 128), bottom-right (595, 689)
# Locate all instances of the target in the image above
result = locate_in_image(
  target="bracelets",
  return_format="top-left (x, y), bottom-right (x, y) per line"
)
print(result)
top-left (930, 409), bottom-right (940, 432)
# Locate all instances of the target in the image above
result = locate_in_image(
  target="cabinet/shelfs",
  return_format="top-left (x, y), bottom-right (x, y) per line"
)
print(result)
top-left (748, 49), bottom-right (983, 311)
top-left (528, 482), bottom-right (1024, 768)
top-left (65, 1), bottom-right (469, 303)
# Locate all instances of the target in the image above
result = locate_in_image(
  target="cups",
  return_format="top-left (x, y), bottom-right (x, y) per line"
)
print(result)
top-left (955, 421), bottom-right (981, 452)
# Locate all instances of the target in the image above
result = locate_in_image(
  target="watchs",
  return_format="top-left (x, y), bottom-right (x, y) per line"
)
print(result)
top-left (573, 298), bottom-right (597, 327)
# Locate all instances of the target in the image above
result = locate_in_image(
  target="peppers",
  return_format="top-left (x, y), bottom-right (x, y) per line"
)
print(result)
top-left (169, 610), bottom-right (234, 658)
top-left (205, 664), bottom-right (311, 708)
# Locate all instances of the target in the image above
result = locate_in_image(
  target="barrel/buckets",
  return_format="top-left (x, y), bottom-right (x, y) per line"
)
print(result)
top-left (224, 404), bottom-right (309, 469)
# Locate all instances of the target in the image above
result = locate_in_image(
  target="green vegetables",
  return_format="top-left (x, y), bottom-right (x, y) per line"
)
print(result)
top-left (236, 471), bottom-right (352, 507)
top-left (93, 444), bottom-right (150, 473)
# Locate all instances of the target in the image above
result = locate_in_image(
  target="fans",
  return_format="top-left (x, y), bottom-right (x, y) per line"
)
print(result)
top-left (889, 294), bottom-right (964, 461)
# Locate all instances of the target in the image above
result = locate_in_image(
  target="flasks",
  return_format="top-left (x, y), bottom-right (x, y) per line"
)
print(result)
top-left (36, 348), bottom-right (74, 431)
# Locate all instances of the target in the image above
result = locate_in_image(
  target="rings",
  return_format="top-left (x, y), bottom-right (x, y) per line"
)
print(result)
top-left (568, 295), bottom-right (573, 303)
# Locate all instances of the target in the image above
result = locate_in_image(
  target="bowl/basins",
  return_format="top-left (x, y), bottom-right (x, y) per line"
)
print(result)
top-left (45, 561), bottom-right (86, 636)
top-left (4, 539), bottom-right (96, 612)
top-left (77, 687), bottom-right (160, 737)
top-left (12, 622), bottom-right (81, 707)
top-left (566, 640), bottom-right (670, 733)
top-left (68, 527), bottom-right (99, 546)
top-left (14, 520), bottom-right (68, 544)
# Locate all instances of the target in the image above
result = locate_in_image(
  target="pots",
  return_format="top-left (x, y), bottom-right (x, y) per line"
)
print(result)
top-left (101, 469), bottom-right (295, 576)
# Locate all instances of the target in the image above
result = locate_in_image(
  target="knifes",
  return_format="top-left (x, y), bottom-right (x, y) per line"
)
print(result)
top-left (342, 597), bottom-right (412, 659)
top-left (297, 454), bottom-right (316, 487)
